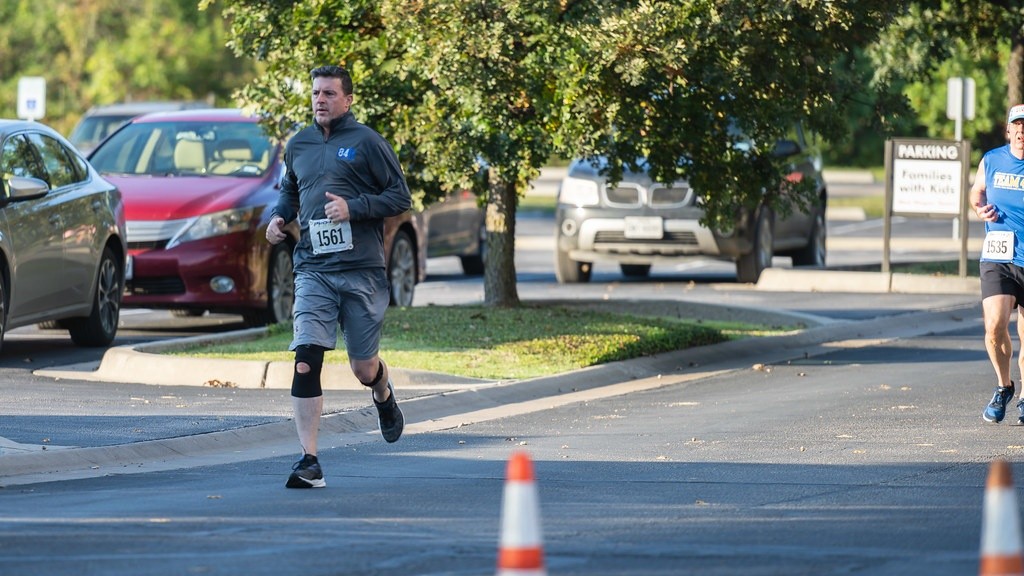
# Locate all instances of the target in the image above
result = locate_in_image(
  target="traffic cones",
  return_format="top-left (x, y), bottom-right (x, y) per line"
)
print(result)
top-left (980, 458), bottom-right (1024, 576)
top-left (497, 452), bottom-right (546, 575)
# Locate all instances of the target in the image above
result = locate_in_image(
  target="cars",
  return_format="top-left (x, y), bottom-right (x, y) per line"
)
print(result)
top-left (425, 152), bottom-right (488, 277)
top-left (67, 100), bottom-right (224, 174)
top-left (0, 119), bottom-right (129, 355)
top-left (39, 109), bottom-right (430, 331)
top-left (555, 92), bottom-right (827, 284)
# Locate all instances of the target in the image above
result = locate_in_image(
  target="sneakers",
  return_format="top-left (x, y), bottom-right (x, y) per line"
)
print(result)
top-left (1016, 398), bottom-right (1024, 425)
top-left (982, 380), bottom-right (1015, 422)
top-left (286, 445), bottom-right (326, 490)
top-left (372, 377), bottom-right (406, 444)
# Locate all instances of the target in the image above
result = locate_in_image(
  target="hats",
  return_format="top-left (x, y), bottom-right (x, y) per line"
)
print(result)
top-left (1008, 104), bottom-right (1024, 123)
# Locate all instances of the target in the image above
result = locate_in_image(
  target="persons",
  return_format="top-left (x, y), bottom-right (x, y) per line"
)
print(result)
top-left (967, 104), bottom-right (1023, 427)
top-left (266, 66), bottom-right (412, 490)
